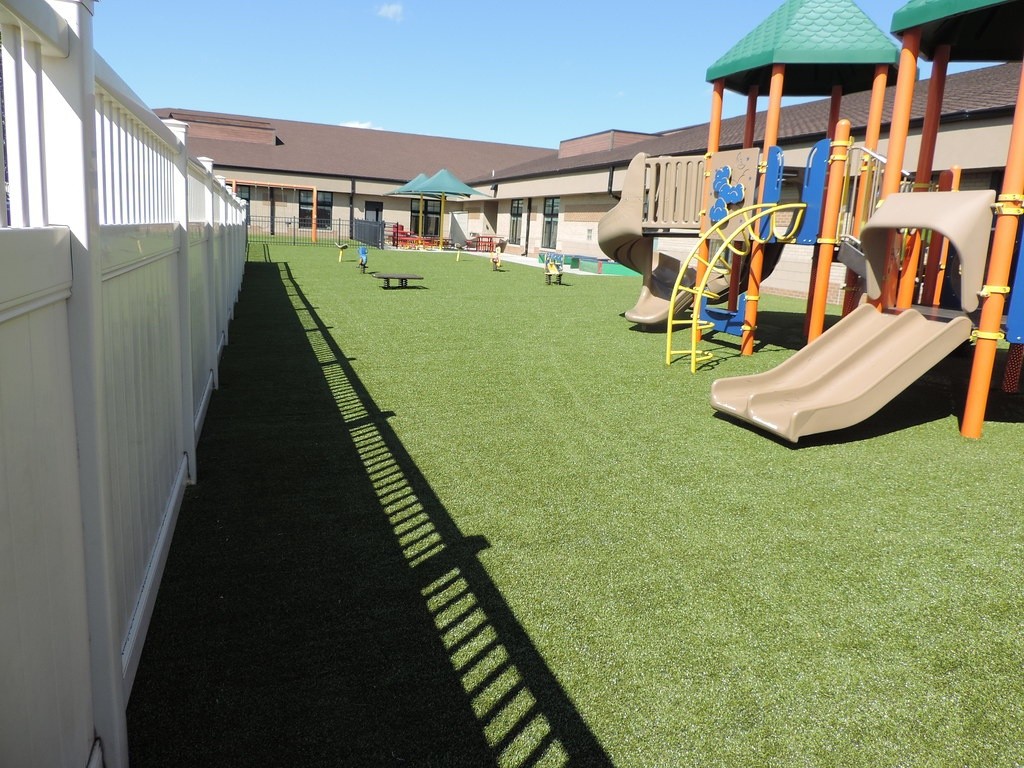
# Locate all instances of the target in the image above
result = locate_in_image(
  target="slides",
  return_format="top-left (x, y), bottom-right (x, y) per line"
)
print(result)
top-left (598, 152), bottom-right (698, 324)
top-left (710, 303), bottom-right (975, 444)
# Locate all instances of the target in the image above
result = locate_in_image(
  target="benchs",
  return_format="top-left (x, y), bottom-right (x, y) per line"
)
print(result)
top-left (464, 240), bottom-right (497, 254)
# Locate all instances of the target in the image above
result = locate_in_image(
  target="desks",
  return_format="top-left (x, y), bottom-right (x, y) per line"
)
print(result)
top-left (476, 235), bottom-right (504, 252)
top-left (401, 237), bottom-right (453, 250)
